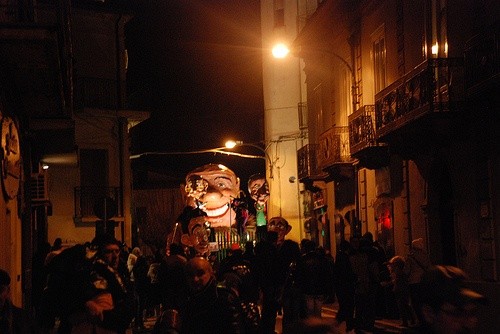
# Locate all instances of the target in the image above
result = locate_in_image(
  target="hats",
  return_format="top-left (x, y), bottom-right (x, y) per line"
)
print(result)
top-left (412, 265), bottom-right (490, 306)
top-left (98, 233), bottom-right (122, 246)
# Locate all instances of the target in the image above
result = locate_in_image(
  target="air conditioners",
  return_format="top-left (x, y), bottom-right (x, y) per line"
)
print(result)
top-left (29, 172), bottom-right (49, 202)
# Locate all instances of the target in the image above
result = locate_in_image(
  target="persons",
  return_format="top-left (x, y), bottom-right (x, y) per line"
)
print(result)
top-left (239, 220), bottom-right (432, 334)
top-left (180, 163), bottom-right (241, 230)
top-left (267, 217), bottom-right (292, 243)
top-left (178, 205), bottom-right (211, 254)
top-left (0, 268), bottom-right (45, 334)
top-left (34, 237), bottom-right (240, 334)
top-left (247, 174), bottom-right (271, 226)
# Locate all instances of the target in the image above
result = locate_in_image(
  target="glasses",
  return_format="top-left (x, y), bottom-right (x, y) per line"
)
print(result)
top-left (439, 308), bottom-right (481, 317)
